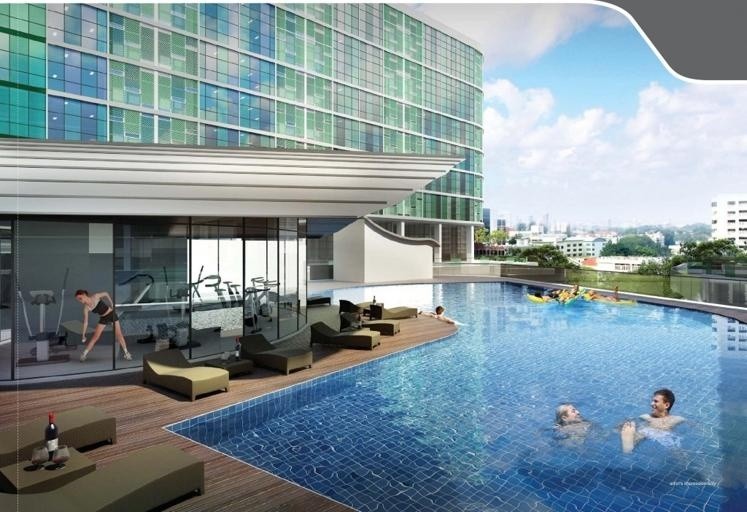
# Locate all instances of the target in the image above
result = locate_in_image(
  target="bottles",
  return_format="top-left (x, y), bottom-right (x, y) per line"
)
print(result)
top-left (45, 411), bottom-right (58, 461)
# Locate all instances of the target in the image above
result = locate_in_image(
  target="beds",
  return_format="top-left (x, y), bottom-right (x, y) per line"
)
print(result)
top-left (311, 321), bottom-right (383, 351)
top-left (142, 347), bottom-right (229, 402)
top-left (339, 299), bottom-right (418, 337)
top-left (0, 404), bottom-right (205, 512)
top-left (236, 334), bottom-right (313, 375)
top-left (307, 296), bottom-right (331, 306)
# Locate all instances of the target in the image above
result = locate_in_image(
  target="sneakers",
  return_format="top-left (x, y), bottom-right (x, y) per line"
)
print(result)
top-left (80, 351), bottom-right (86, 362)
top-left (121, 350), bottom-right (133, 360)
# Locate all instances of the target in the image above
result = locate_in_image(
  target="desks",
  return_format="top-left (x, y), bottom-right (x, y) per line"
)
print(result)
top-left (204, 356), bottom-right (254, 380)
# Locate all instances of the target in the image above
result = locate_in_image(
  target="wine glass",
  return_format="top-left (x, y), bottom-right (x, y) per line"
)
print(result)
top-left (31, 447), bottom-right (50, 472)
top-left (53, 446), bottom-right (72, 471)
